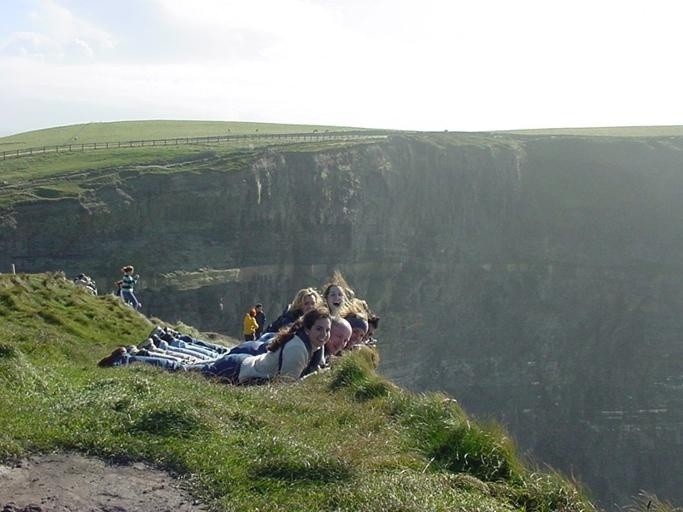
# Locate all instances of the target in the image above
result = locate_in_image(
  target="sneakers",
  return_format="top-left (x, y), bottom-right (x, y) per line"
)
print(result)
top-left (97, 327), bottom-right (177, 366)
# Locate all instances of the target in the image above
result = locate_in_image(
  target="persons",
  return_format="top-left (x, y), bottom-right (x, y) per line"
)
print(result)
top-left (97, 271), bottom-right (379, 388)
top-left (73, 272), bottom-right (96, 295)
top-left (113, 265), bottom-right (143, 311)
